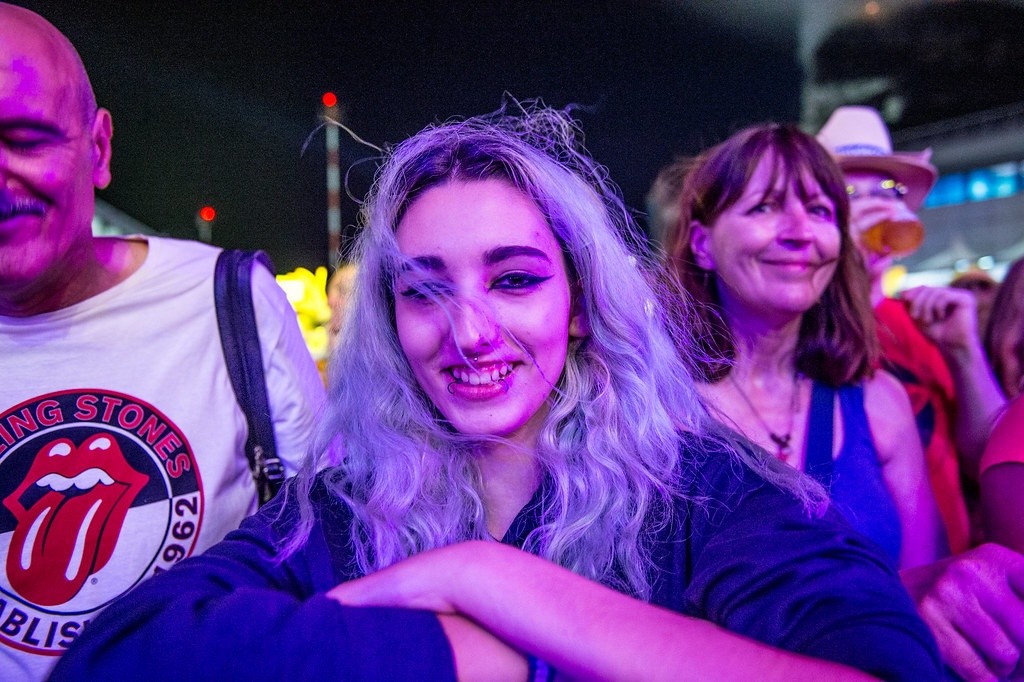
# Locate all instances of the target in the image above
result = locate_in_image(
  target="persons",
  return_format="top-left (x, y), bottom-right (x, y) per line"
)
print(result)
top-left (645, 104), bottom-right (1022, 682)
top-left (43, 87), bottom-right (949, 682)
top-left (0, 0), bottom-right (333, 682)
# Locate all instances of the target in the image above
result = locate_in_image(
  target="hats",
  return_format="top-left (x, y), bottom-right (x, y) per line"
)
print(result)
top-left (814, 105), bottom-right (937, 212)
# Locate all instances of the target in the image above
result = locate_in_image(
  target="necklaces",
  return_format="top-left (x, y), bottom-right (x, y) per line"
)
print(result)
top-left (727, 371), bottom-right (802, 457)
